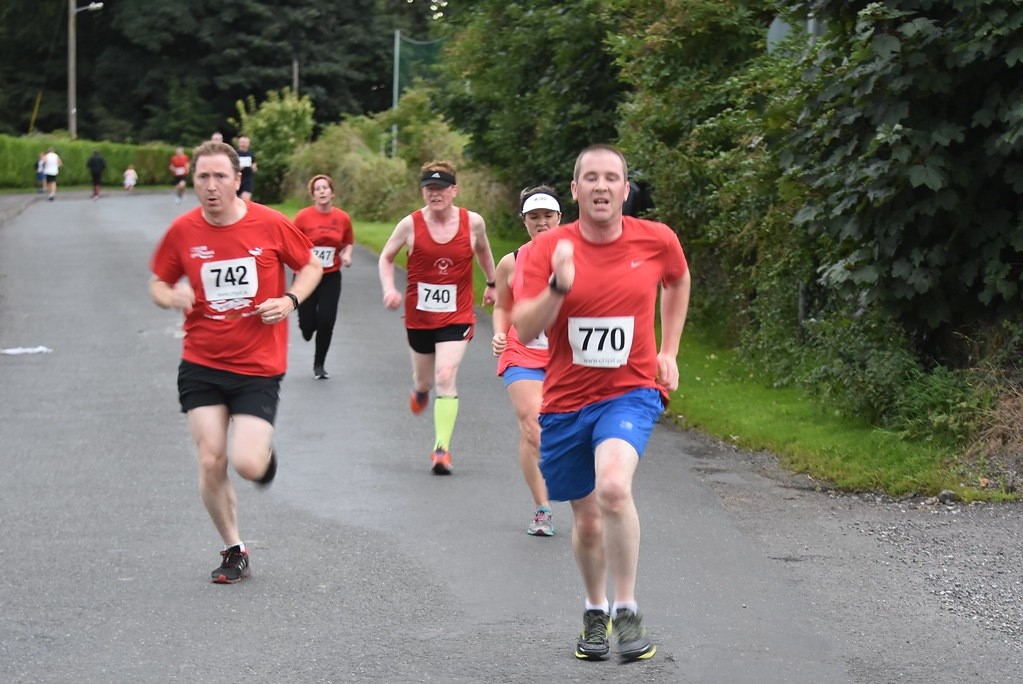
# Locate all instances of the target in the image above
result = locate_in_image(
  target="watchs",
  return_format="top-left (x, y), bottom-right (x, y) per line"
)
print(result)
top-left (487, 281), bottom-right (496, 287)
top-left (547, 272), bottom-right (572, 296)
top-left (286, 292), bottom-right (299, 311)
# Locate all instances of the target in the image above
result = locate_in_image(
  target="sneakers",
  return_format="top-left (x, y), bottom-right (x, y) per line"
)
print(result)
top-left (256, 454), bottom-right (277, 485)
top-left (313, 366), bottom-right (328, 379)
top-left (410, 389), bottom-right (430, 415)
top-left (212, 544), bottom-right (252, 583)
top-left (526, 505), bottom-right (555, 537)
top-left (575, 609), bottom-right (613, 660)
top-left (431, 441), bottom-right (453, 475)
top-left (610, 607), bottom-right (656, 664)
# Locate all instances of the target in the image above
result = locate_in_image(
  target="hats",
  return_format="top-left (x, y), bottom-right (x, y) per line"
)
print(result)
top-left (420, 170), bottom-right (455, 187)
top-left (520, 192), bottom-right (561, 216)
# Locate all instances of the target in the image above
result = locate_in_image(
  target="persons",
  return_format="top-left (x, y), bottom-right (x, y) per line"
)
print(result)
top-left (148, 141), bottom-right (323, 584)
top-left (169, 146), bottom-right (190, 204)
top-left (490, 186), bottom-right (562, 537)
top-left (42, 146), bottom-right (62, 201)
top-left (509, 145), bottom-right (690, 665)
top-left (34, 151), bottom-right (47, 193)
top-left (377, 160), bottom-right (495, 477)
top-left (213, 133), bottom-right (223, 143)
top-left (86, 150), bottom-right (107, 200)
top-left (290, 174), bottom-right (356, 380)
top-left (236, 136), bottom-right (257, 200)
top-left (123, 164), bottom-right (138, 190)
